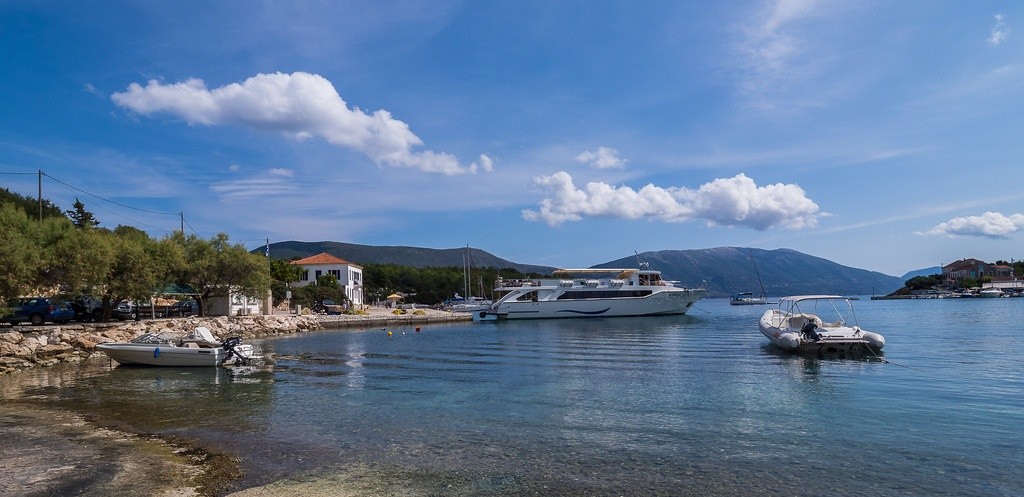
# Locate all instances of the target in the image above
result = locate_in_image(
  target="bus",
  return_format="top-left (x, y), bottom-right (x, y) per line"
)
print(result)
top-left (149, 292), bottom-right (201, 306)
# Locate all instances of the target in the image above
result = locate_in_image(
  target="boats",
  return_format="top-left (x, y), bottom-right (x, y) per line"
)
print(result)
top-left (480, 269), bottom-right (708, 319)
top-left (729, 292), bottom-right (766, 304)
top-left (980, 286), bottom-right (1004, 298)
top-left (94, 327), bottom-right (240, 366)
top-left (758, 295), bottom-right (885, 349)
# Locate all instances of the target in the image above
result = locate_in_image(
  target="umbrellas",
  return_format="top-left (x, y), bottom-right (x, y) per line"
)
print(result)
top-left (387, 294), bottom-right (401, 308)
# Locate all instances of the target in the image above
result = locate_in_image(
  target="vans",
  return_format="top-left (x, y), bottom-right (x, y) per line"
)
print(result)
top-left (0, 297), bottom-right (75, 326)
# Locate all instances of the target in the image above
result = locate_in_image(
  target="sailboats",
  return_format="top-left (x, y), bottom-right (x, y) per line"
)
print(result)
top-left (449, 244), bottom-right (493, 311)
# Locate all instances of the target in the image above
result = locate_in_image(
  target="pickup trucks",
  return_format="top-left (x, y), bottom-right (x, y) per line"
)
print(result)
top-left (313, 300), bottom-right (345, 315)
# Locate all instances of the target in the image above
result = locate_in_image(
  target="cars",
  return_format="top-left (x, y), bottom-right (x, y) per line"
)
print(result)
top-left (60, 294), bottom-right (199, 325)
top-left (957, 288), bottom-right (966, 293)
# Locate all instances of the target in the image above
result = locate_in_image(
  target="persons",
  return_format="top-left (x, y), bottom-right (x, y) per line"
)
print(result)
top-left (804, 318), bottom-right (818, 340)
